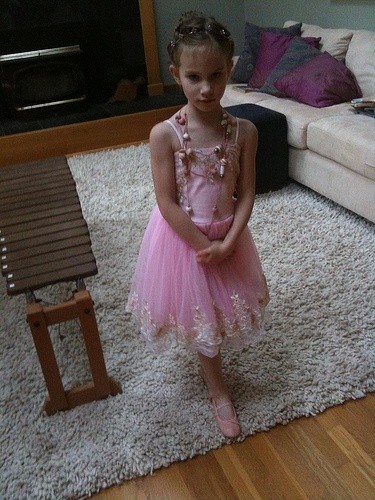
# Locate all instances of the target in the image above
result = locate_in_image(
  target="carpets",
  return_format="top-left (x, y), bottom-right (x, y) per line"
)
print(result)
top-left (0, 141), bottom-right (375, 500)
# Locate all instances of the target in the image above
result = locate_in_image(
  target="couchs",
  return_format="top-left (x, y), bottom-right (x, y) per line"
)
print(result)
top-left (219, 20), bottom-right (375, 225)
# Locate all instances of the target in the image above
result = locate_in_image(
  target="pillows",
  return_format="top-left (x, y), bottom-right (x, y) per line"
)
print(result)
top-left (230, 21), bottom-right (304, 83)
top-left (258, 34), bottom-right (324, 100)
top-left (247, 30), bottom-right (323, 89)
top-left (273, 49), bottom-right (364, 109)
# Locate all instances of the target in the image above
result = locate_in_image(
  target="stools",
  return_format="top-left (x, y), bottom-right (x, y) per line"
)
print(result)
top-left (222, 101), bottom-right (292, 197)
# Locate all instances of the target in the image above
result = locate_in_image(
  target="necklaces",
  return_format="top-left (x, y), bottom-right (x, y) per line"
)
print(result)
top-left (175, 106), bottom-right (239, 214)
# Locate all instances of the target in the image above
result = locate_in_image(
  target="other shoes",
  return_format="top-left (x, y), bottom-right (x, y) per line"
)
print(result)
top-left (210, 393), bottom-right (240, 439)
top-left (198, 367), bottom-right (229, 395)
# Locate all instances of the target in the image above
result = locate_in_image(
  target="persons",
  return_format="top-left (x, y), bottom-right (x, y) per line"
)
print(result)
top-left (126, 10), bottom-right (270, 440)
